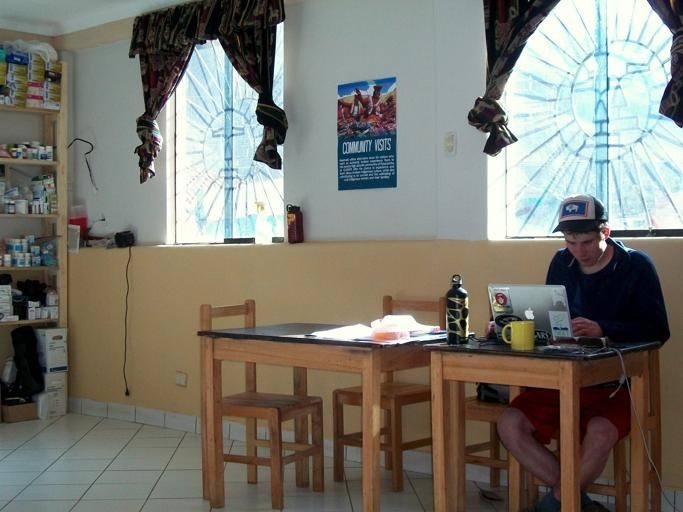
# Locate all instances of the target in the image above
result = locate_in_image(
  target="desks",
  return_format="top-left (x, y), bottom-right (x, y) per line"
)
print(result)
top-left (197, 321), bottom-right (477, 511)
top-left (423, 339), bottom-right (659, 512)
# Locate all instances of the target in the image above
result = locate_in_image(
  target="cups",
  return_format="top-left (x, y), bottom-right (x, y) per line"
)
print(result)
top-left (501, 321), bottom-right (536, 352)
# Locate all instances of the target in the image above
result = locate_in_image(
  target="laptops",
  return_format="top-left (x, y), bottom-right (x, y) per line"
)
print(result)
top-left (488, 283), bottom-right (572, 340)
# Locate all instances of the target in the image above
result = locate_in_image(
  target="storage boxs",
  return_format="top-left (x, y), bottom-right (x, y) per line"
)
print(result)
top-left (37, 327), bottom-right (68, 374)
top-left (1, 401), bottom-right (37, 422)
top-left (44, 374), bottom-right (67, 416)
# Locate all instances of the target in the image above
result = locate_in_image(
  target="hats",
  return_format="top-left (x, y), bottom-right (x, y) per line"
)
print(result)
top-left (552, 195), bottom-right (608, 233)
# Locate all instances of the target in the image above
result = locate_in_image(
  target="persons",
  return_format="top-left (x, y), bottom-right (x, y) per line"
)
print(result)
top-left (336, 83), bottom-right (396, 135)
top-left (480, 194), bottom-right (672, 512)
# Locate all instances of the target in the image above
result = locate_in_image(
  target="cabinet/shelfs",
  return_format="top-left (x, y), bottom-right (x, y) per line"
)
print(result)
top-left (1, 60), bottom-right (69, 420)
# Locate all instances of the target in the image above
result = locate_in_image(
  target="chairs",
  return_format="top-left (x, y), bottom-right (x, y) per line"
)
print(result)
top-left (197, 301), bottom-right (325, 511)
top-left (332, 295), bottom-right (448, 492)
top-left (463, 395), bottom-right (508, 504)
top-left (527, 430), bottom-right (624, 511)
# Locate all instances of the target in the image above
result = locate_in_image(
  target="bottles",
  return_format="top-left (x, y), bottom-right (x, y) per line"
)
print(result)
top-left (1, 140), bottom-right (53, 267)
top-left (445, 274), bottom-right (469, 344)
top-left (285, 204), bottom-right (303, 244)
top-left (35, 306), bottom-right (48, 320)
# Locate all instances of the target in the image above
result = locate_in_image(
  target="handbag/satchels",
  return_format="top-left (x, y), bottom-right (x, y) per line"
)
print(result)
top-left (475, 384), bottom-right (526, 404)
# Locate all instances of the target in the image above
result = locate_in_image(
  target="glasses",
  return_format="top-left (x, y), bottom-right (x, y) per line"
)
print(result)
top-left (554, 336), bottom-right (602, 348)
